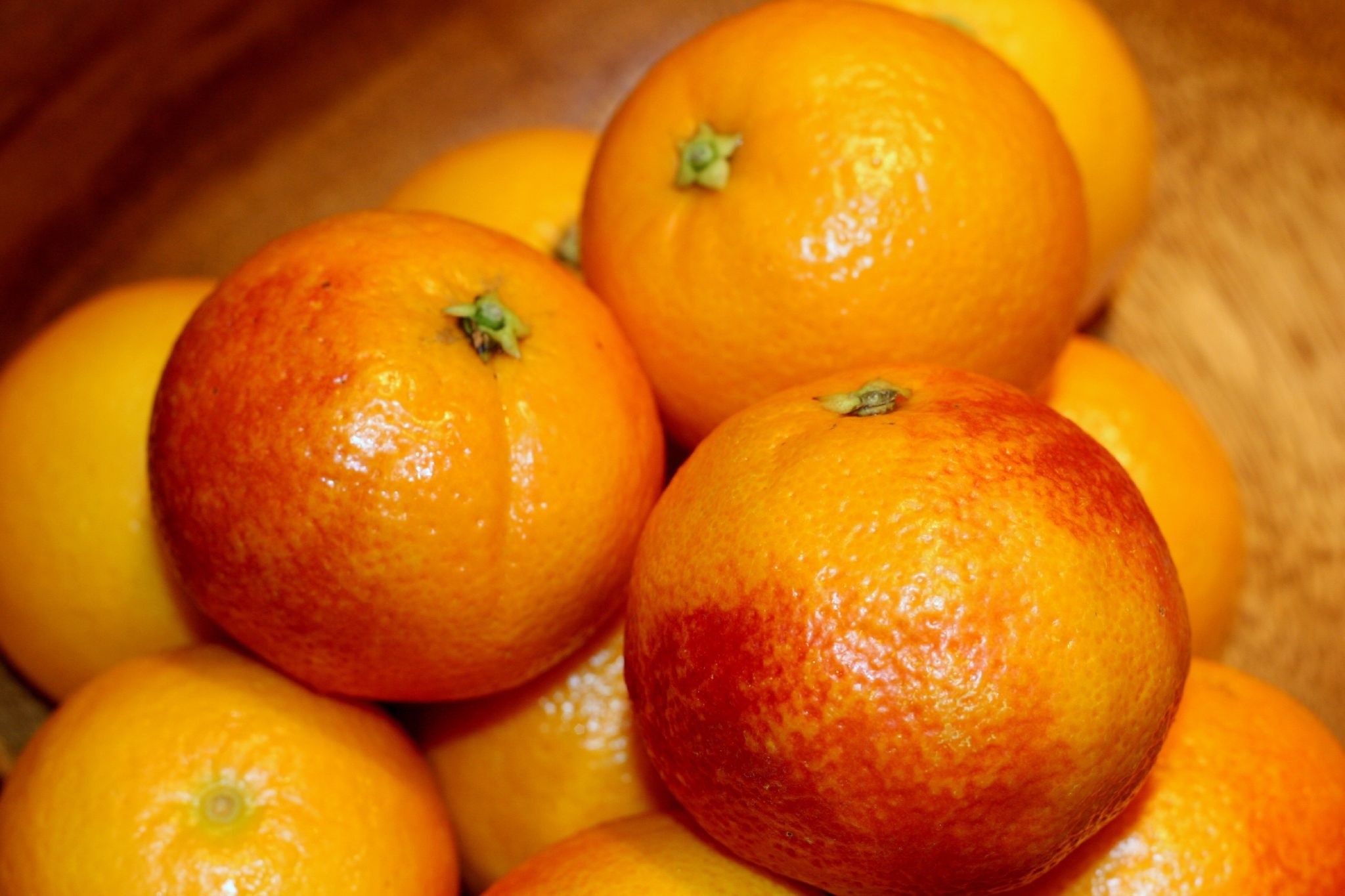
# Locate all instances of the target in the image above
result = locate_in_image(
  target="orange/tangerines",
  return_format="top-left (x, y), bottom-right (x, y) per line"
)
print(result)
top-left (0, 0), bottom-right (1345, 896)
